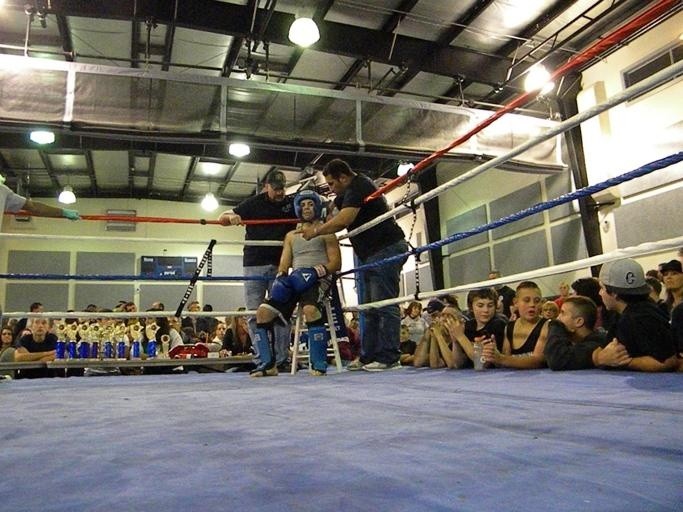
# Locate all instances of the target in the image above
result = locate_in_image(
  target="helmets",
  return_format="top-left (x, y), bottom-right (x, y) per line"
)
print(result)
top-left (293, 189), bottom-right (323, 220)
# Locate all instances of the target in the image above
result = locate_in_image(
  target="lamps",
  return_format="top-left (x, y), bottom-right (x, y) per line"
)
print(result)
top-left (396, 159), bottom-right (414, 177)
top-left (286, 6), bottom-right (322, 48)
top-left (28, 129), bottom-right (56, 145)
top-left (228, 144), bottom-right (251, 159)
top-left (57, 174), bottom-right (77, 204)
top-left (523, 63), bottom-right (554, 97)
top-left (200, 176), bottom-right (219, 212)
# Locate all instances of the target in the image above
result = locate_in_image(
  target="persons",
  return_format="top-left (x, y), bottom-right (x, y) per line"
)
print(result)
top-left (219, 171), bottom-right (305, 378)
top-left (0, 300), bottom-right (259, 380)
top-left (292, 158), bottom-right (408, 372)
top-left (400, 258), bottom-right (682, 372)
top-left (249, 190), bottom-right (341, 378)
top-left (277, 312), bottom-right (360, 372)
top-left (0, 179), bottom-right (80, 232)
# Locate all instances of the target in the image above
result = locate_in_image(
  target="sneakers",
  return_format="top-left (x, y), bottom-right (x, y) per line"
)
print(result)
top-left (250, 361), bottom-right (300, 376)
top-left (345, 357), bottom-right (403, 372)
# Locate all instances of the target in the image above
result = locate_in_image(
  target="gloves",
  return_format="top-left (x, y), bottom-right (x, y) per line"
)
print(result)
top-left (270, 264), bottom-right (329, 304)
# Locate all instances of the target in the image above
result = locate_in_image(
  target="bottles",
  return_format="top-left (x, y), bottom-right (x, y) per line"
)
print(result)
top-left (472, 337), bottom-right (484, 369)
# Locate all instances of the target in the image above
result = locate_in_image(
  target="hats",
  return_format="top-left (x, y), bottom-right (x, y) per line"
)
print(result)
top-left (660, 259), bottom-right (683, 274)
top-left (426, 297), bottom-right (446, 314)
top-left (265, 170), bottom-right (287, 190)
top-left (598, 256), bottom-right (651, 297)
